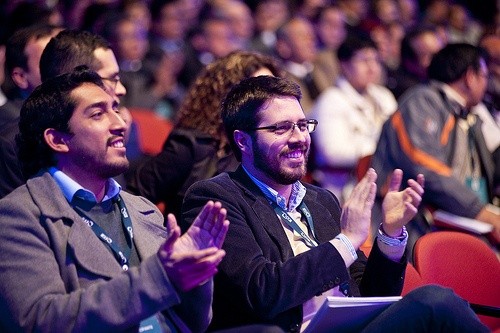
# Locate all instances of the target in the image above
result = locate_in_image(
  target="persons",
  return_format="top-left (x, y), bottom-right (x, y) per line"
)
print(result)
top-left (0, 0), bottom-right (500, 332)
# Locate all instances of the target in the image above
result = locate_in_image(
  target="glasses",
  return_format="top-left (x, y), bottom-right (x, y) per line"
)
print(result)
top-left (99, 78), bottom-right (120, 91)
top-left (246, 119), bottom-right (318, 135)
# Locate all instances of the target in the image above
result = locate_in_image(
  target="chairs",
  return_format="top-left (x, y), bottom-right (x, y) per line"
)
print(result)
top-left (356, 156), bottom-right (499, 241)
top-left (358, 246), bottom-right (422, 333)
top-left (413, 231), bottom-right (500, 333)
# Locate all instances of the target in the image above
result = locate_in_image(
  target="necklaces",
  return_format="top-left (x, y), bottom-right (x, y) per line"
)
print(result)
top-left (266, 195), bottom-right (322, 248)
